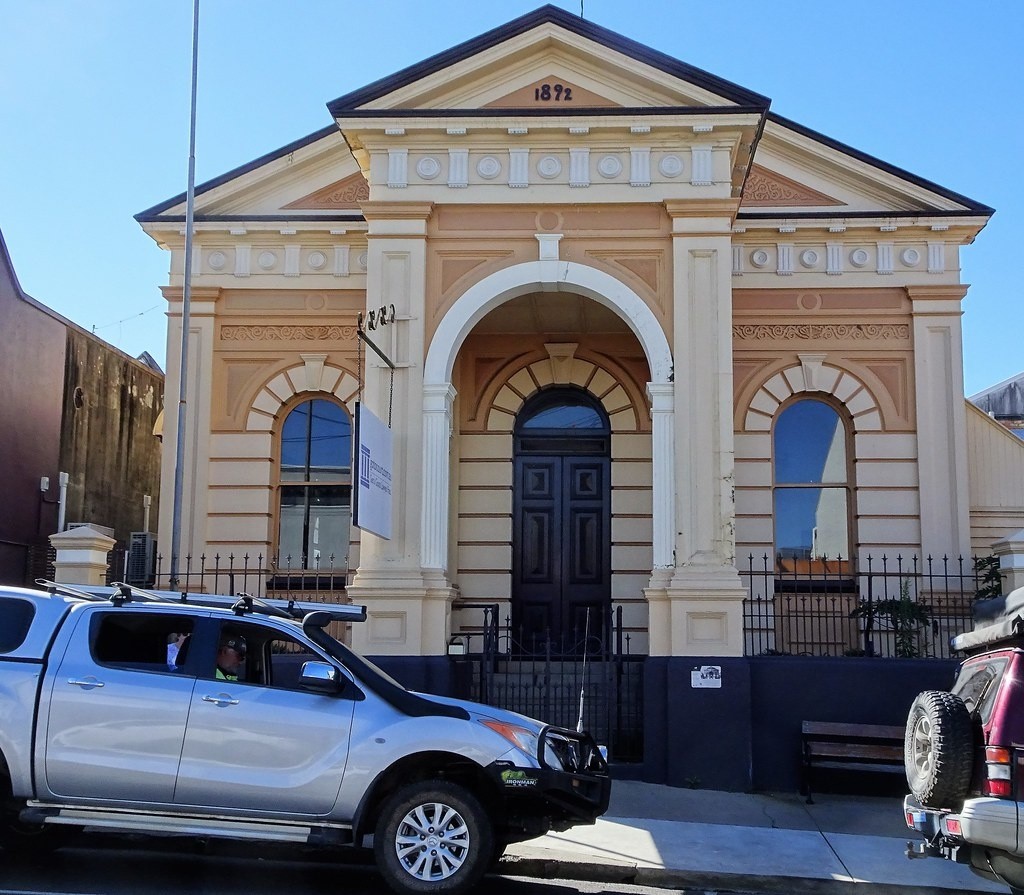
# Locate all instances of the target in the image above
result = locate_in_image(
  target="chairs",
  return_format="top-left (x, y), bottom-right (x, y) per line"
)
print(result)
top-left (171, 635), bottom-right (191, 674)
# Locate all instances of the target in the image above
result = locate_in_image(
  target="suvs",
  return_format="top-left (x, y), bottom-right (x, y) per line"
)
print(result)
top-left (902, 584), bottom-right (1023, 894)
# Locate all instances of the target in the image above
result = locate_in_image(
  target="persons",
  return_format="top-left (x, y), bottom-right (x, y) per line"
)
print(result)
top-left (216, 637), bottom-right (247, 682)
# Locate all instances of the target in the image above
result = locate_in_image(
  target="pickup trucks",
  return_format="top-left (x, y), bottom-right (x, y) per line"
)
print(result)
top-left (0, 575), bottom-right (613, 894)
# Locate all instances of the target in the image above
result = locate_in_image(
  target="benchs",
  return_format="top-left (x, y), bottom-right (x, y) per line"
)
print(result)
top-left (799, 719), bottom-right (905, 808)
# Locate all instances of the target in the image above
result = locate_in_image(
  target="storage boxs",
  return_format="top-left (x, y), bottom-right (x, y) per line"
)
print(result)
top-left (447, 636), bottom-right (468, 655)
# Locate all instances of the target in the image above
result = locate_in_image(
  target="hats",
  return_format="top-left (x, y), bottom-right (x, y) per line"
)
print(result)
top-left (221, 633), bottom-right (247, 658)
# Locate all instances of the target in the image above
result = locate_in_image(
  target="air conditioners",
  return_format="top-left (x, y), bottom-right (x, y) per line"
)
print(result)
top-left (66, 522), bottom-right (115, 538)
top-left (127, 531), bottom-right (158, 582)
